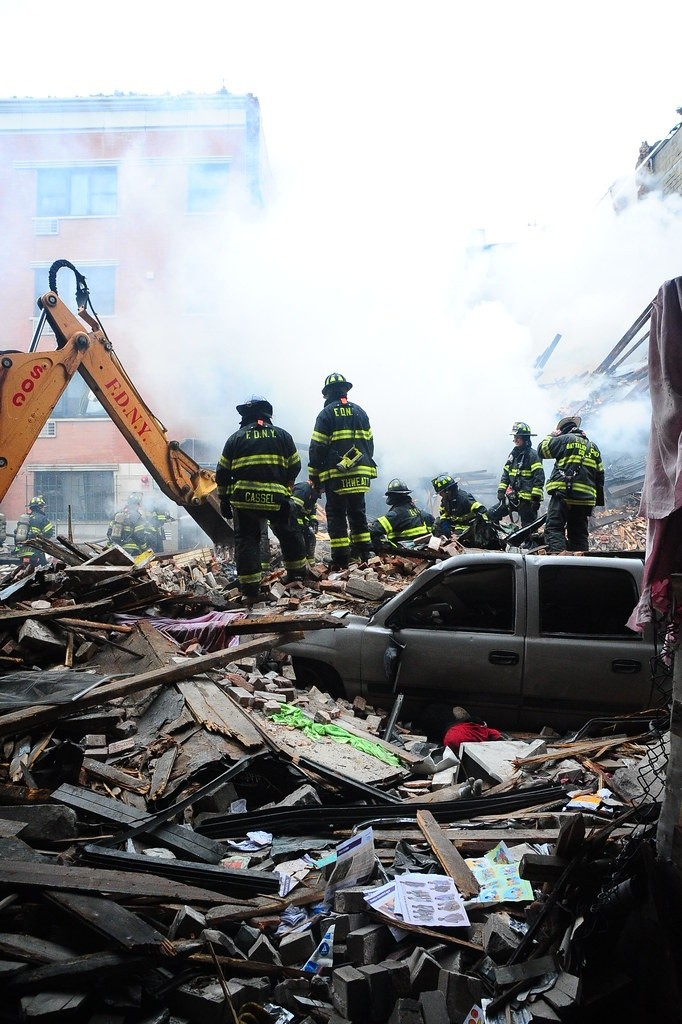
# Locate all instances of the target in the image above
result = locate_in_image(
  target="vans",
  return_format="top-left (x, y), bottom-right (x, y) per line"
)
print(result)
top-left (273, 549), bottom-right (670, 742)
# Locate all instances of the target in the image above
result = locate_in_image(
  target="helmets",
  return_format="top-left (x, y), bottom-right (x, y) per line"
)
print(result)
top-left (322, 373), bottom-right (352, 395)
top-left (236, 394), bottom-right (273, 417)
top-left (557, 417), bottom-right (581, 431)
top-left (433, 476), bottom-right (460, 494)
top-left (509, 422), bottom-right (537, 436)
top-left (385, 478), bottom-right (414, 495)
top-left (25, 497), bottom-right (48, 507)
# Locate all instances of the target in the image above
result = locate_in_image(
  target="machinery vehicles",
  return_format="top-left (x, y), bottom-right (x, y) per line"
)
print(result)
top-left (0, 259), bottom-right (282, 570)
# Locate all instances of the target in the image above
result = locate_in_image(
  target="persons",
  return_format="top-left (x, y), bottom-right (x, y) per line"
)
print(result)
top-left (215, 394), bottom-right (308, 597)
top-left (488, 421), bottom-right (545, 542)
top-left (107, 491), bottom-right (176, 556)
top-left (308, 372), bottom-right (377, 568)
top-left (370, 477), bottom-right (435, 557)
top-left (260, 518), bottom-right (270, 574)
top-left (431, 474), bottom-right (506, 549)
top-left (14, 495), bottom-right (55, 569)
top-left (537, 417), bottom-right (606, 556)
top-left (267, 480), bottom-right (325, 569)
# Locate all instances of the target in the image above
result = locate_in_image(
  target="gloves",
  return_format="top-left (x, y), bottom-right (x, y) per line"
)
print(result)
top-left (220, 494), bottom-right (233, 519)
top-left (498, 491), bottom-right (505, 501)
top-left (530, 500), bottom-right (540, 511)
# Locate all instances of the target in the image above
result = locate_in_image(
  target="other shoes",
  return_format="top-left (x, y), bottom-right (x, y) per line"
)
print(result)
top-left (241, 583), bottom-right (260, 596)
